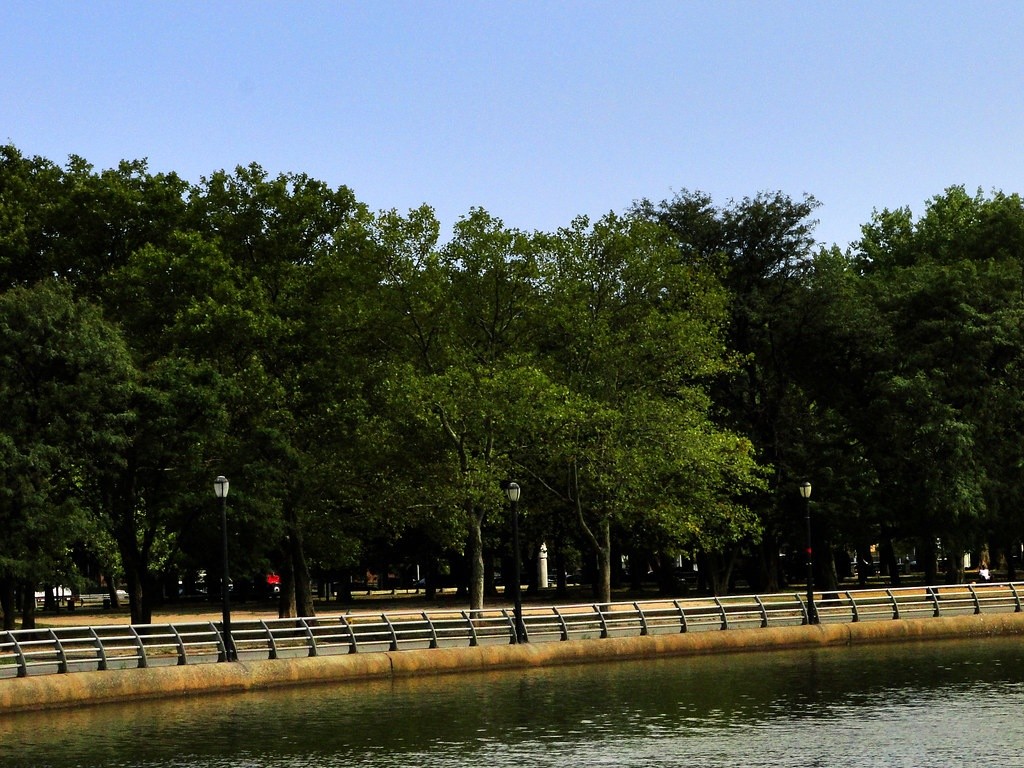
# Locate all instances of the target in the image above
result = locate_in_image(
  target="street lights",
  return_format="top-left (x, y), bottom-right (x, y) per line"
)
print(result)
top-left (799, 481), bottom-right (817, 624)
top-left (213, 474), bottom-right (236, 663)
top-left (507, 482), bottom-right (526, 644)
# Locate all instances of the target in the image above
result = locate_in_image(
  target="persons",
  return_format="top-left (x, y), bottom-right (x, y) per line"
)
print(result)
top-left (267, 572), bottom-right (280, 584)
top-left (413, 576), bottom-right (416, 589)
top-left (420, 577), bottom-right (425, 588)
top-left (979, 559), bottom-right (997, 571)
top-left (81, 598), bottom-right (83, 607)
